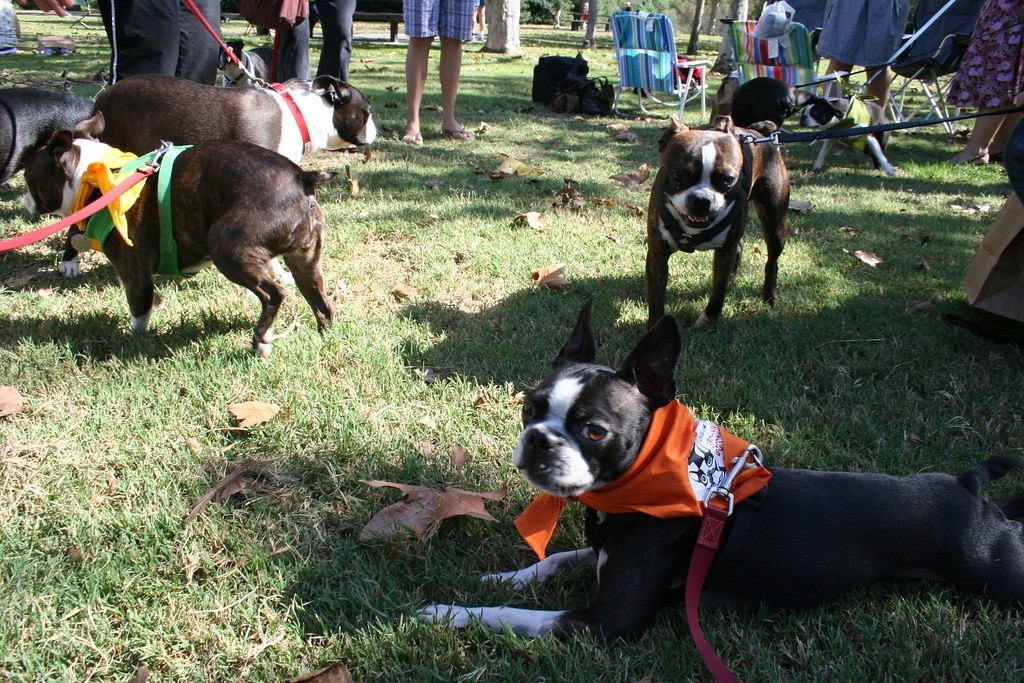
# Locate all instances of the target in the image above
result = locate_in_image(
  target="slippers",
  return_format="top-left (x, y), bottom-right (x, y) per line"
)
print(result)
top-left (402, 132), bottom-right (423, 144)
top-left (442, 127), bottom-right (475, 139)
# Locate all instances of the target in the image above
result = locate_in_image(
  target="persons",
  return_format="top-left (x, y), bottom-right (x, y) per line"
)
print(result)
top-left (471, 0), bottom-right (487, 42)
top-left (402, 0), bottom-right (475, 144)
top-left (625, 2), bottom-right (630, 11)
top-left (553, 0), bottom-right (561, 30)
top-left (816, 0), bottom-right (910, 112)
top-left (276, 0), bottom-right (357, 83)
top-left (17, 0), bottom-right (221, 87)
top-left (0, 0), bottom-right (21, 55)
top-left (944, 0), bottom-right (1024, 166)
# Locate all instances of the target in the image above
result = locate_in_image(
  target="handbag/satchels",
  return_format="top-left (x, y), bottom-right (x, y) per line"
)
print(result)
top-left (532, 57), bottom-right (614, 115)
top-left (750, 1), bottom-right (795, 37)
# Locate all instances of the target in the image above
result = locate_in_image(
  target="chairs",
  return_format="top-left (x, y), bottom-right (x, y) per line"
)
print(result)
top-left (887, 0), bottom-right (985, 137)
top-left (720, 18), bottom-right (851, 99)
top-left (609, 10), bottom-right (712, 123)
top-left (62, 0), bottom-right (91, 29)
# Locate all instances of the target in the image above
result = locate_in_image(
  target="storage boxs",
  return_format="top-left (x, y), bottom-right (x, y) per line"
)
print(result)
top-left (37, 34), bottom-right (76, 55)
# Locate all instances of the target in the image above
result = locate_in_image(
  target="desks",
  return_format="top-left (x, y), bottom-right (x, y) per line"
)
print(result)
top-left (567, 12), bottom-right (611, 31)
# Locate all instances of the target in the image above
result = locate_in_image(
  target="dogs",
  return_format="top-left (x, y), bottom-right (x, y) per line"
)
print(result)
top-left (731, 77), bottom-right (895, 176)
top-left (0, 39), bottom-right (378, 361)
top-left (643, 114), bottom-right (792, 330)
top-left (418, 300), bottom-right (1024, 650)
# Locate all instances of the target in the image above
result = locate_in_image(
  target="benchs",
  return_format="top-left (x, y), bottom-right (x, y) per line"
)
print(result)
top-left (565, 20), bottom-right (611, 25)
top-left (352, 11), bottom-right (405, 43)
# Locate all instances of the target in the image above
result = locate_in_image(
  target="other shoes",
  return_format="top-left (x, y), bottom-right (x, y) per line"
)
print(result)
top-left (963, 153), bottom-right (989, 166)
